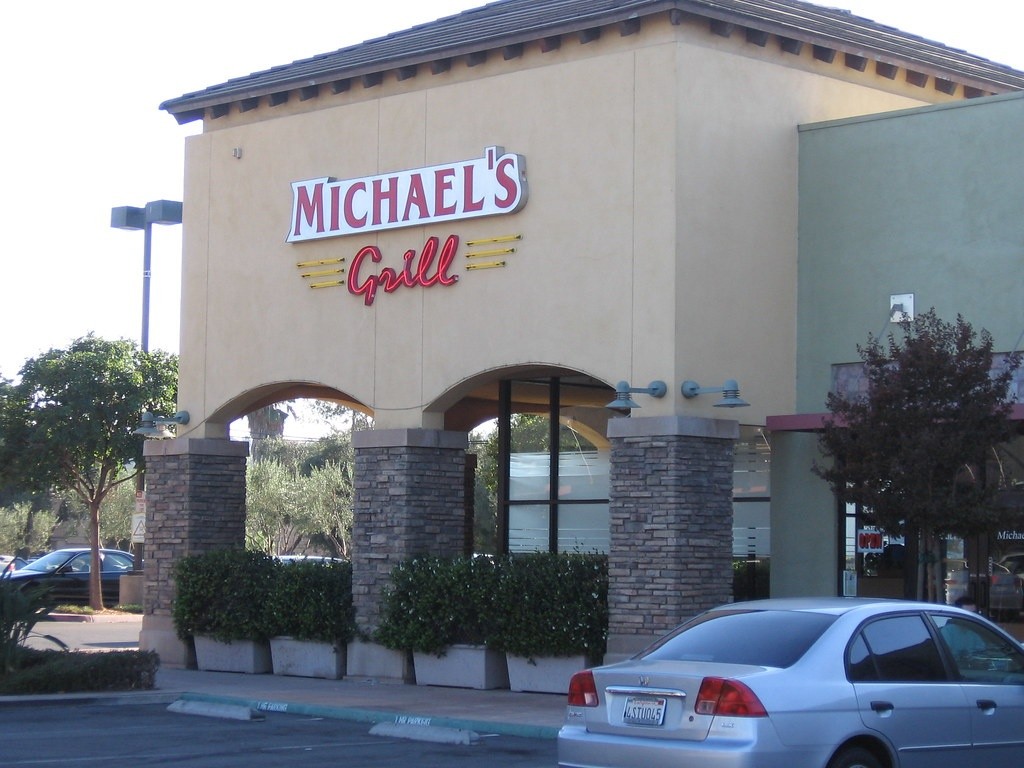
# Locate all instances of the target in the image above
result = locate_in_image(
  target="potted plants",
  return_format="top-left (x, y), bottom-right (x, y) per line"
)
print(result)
top-left (358, 542), bottom-right (611, 695)
top-left (167, 545), bottom-right (357, 680)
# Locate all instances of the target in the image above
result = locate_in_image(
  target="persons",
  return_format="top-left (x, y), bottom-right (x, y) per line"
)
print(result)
top-left (940, 598), bottom-right (987, 670)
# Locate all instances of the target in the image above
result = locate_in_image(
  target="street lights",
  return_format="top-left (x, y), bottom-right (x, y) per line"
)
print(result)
top-left (112, 200), bottom-right (188, 575)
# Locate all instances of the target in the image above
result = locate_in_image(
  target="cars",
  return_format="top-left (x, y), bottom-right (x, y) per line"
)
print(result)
top-left (0, 556), bottom-right (30, 577)
top-left (944, 559), bottom-right (1024, 621)
top-left (554, 595), bottom-right (1023, 768)
top-left (0, 547), bottom-right (145, 611)
top-left (999, 553), bottom-right (1024, 589)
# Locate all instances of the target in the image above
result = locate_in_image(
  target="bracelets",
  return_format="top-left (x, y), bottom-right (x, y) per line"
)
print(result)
top-left (968, 662), bottom-right (971, 669)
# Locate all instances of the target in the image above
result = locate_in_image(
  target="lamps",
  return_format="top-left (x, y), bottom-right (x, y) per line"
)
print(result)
top-left (681, 378), bottom-right (751, 408)
top-left (131, 410), bottom-right (190, 437)
top-left (605, 380), bottom-right (667, 410)
